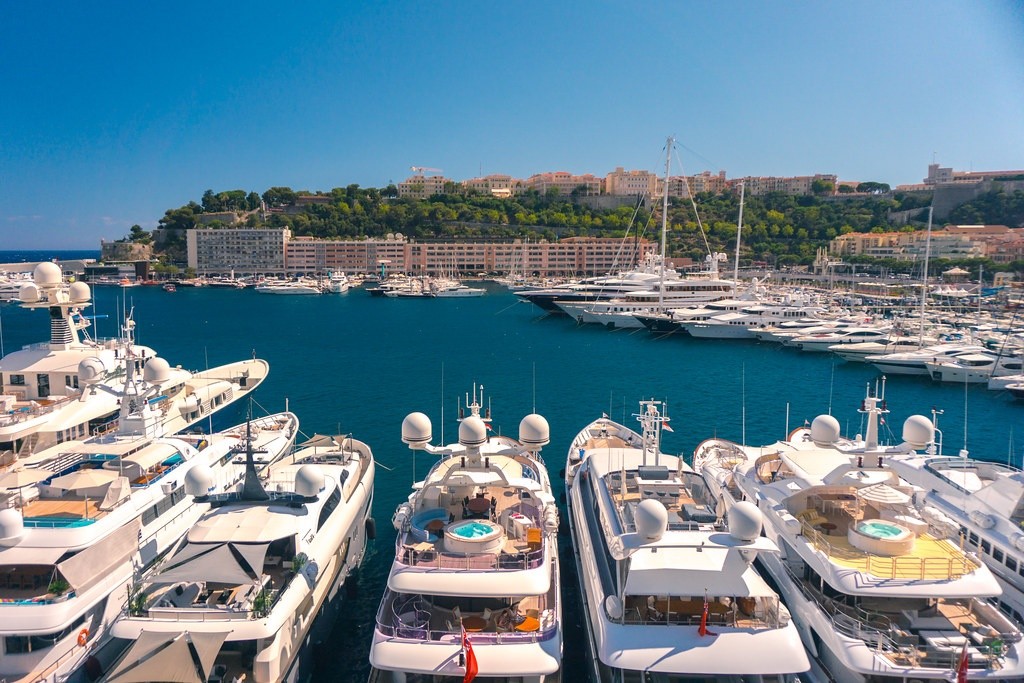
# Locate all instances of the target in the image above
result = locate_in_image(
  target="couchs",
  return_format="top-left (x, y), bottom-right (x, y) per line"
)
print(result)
top-left (918, 630), bottom-right (971, 645)
top-left (928, 639), bottom-right (981, 661)
top-left (235, 574), bottom-right (270, 610)
top-left (148, 582), bottom-right (206, 607)
top-left (968, 625), bottom-right (1000, 644)
top-left (891, 623), bottom-right (919, 645)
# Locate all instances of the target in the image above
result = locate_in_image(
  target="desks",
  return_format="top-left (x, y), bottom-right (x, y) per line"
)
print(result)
top-left (820, 522), bottom-right (837, 535)
top-left (468, 498), bottom-right (490, 515)
top-left (148, 465), bottom-right (172, 478)
top-left (512, 616), bottom-right (540, 632)
top-left (958, 623), bottom-right (979, 636)
top-left (265, 556), bottom-right (281, 566)
top-left (461, 617), bottom-right (487, 631)
top-left (131, 473), bottom-right (155, 486)
top-left (656, 600), bottom-right (730, 616)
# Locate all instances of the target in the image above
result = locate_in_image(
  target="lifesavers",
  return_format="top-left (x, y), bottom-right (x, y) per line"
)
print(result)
top-left (77, 629), bottom-right (90, 646)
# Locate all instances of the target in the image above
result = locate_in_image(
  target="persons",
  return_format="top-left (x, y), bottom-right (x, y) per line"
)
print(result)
top-left (498, 610), bottom-right (514, 631)
top-left (509, 603), bottom-right (525, 625)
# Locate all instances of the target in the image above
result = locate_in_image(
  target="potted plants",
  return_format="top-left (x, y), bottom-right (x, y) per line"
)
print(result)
top-left (251, 595), bottom-right (274, 616)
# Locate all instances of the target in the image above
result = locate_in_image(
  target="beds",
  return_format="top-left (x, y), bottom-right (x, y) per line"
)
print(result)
top-left (900, 608), bottom-right (956, 632)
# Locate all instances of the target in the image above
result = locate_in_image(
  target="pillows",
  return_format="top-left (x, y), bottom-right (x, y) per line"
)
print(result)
top-left (174, 585), bottom-right (183, 596)
top-left (180, 582), bottom-right (188, 591)
top-left (977, 624), bottom-right (991, 635)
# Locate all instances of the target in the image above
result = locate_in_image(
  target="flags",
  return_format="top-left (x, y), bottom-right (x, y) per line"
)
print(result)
top-left (462, 624), bottom-right (479, 682)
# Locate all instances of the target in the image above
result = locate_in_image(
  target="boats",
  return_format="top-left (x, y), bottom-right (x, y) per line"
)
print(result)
top-left (0, 134), bottom-right (1024, 683)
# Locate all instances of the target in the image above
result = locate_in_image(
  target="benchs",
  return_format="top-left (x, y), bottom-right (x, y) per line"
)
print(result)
top-left (411, 508), bottom-right (449, 544)
top-left (682, 503), bottom-right (717, 523)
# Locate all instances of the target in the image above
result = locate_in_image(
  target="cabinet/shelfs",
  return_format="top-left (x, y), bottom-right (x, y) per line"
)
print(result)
top-left (500, 509), bottom-right (532, 538)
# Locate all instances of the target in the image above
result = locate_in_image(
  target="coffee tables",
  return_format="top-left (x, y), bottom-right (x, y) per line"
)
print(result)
top-left (216, 588), bottom-right (235, 607)
top-left (191, 588), bottom-right (214, 608)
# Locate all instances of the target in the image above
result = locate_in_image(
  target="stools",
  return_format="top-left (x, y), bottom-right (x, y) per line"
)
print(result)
top-left (657, 492), bottom-right (666, 504)
top-left (670, 492), bottom-right (681, 509)
top-left (644, 489), bottom-right (654, 499)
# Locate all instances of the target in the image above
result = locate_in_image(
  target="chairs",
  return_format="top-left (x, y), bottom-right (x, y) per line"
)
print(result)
top-left (481, 608), bottom-right (492, 624)
top-left (494, 613), bottom-right (511, 634)
top-left (461, 493), bottom-right (497, 519)
top-left (446, 620), bottom-right (460, 632)
top-left (646, 595), bottom-right (739, 627)
top-left (453, 607), bottom-right (471, 622)
top-left (402, 542), bottom-right (434, 565)
top-left (525, 607), bottom-right (541, 619)
